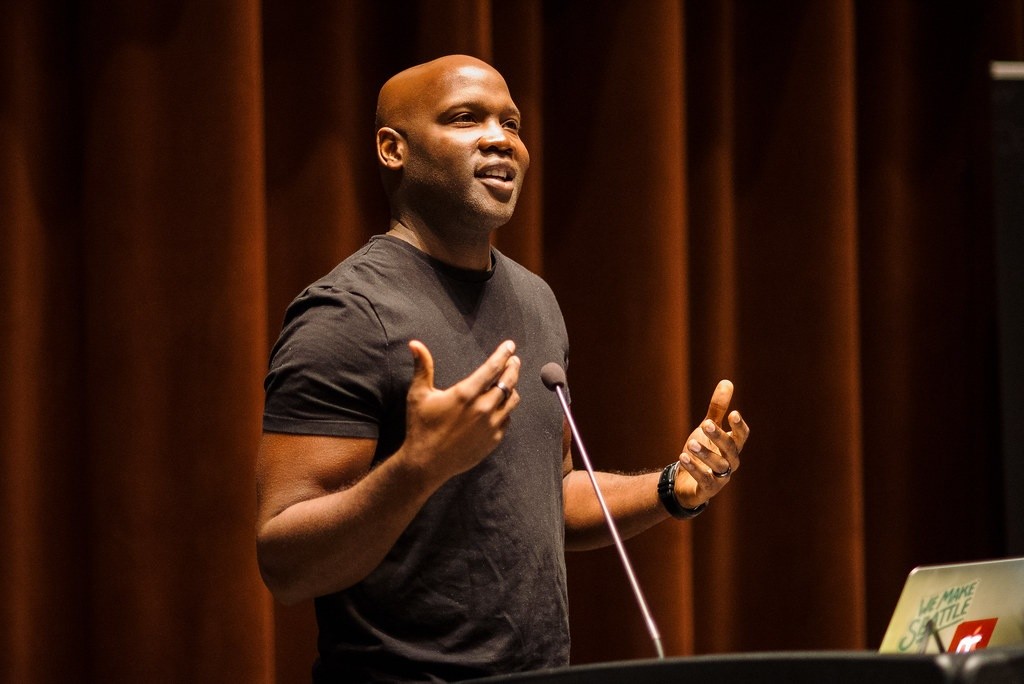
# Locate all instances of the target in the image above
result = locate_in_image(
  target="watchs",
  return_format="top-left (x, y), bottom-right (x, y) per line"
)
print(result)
top-left (658, 462), bottom-right (710, 522)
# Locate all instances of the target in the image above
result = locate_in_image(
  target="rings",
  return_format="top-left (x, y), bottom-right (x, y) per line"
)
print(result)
top-left (490, 382), bottom-right (511, 404)
top-left (712, 467), bottom-right (731, 478)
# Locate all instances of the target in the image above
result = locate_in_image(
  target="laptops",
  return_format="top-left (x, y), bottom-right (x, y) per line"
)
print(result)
top-left (878, 557), bottom-right (1024, 655)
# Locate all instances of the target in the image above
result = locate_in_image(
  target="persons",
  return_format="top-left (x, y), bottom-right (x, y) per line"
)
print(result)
top-left (252, 53), bottom-right (749, 683)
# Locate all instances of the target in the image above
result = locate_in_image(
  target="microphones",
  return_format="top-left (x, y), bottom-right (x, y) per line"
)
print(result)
top-left (539, 362), bottom-right (666, 660)
